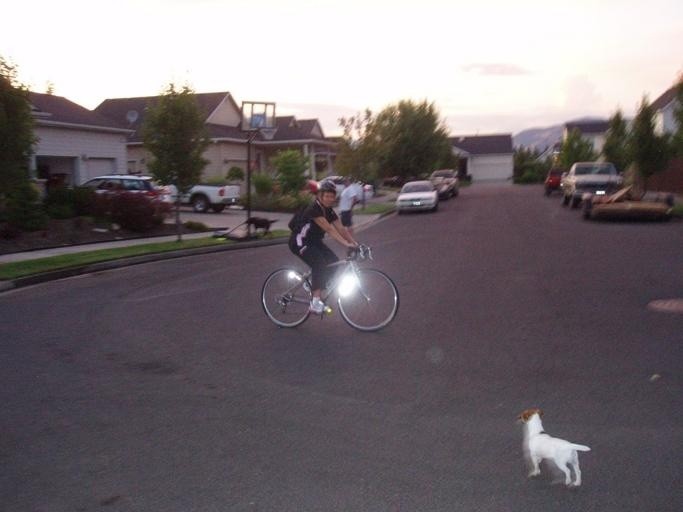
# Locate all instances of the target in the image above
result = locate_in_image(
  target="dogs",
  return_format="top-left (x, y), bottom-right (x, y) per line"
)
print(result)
top-left (246, 217), bottom-right (278, 235)
top-left (514, 408), bottom-right (592, 487)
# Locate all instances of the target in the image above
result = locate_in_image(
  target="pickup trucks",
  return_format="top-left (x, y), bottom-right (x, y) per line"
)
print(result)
top-left (561, 160), bottom-right (626, 209)
top-left (36, 164), bottom-right (68, 194)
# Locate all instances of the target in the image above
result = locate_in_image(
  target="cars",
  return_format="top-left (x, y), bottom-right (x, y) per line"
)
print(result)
top-left (397, 181), bottom-right (440, 213)
top-left (316, 175), bottom-right (376, 203)
top-left (274, 179), bottom-right (321, 194)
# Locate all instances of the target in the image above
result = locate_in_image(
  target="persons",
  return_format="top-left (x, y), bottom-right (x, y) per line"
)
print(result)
top-left (338, 176), bottom-right (360, 258)
top-left (362, 180), bottom-right (373, 211)
top-left (102, 182), bottom-right (114, 194)
top-left (305, 176), bottom-right (317, 195)
top-left (159, 181), bottom-right (171, 204)
top-left (286, 178), bottom-right (360, 315)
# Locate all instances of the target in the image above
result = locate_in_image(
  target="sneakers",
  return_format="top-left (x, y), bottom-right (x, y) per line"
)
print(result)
top-left (307, 300), bottom-right (330, 314)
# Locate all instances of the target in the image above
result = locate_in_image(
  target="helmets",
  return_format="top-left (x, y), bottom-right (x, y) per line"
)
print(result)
top-left (319, 180), bottom-right (336, 192)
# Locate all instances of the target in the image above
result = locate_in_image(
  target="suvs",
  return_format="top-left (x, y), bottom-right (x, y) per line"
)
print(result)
top-left (155, 173), bottom-right (241, 213)
top-left (59, 175), bottom-right (173, 230)
top-left (430, 169), bottom-right (460, 201)
top-left (544, 166), bottom-right (566, 198)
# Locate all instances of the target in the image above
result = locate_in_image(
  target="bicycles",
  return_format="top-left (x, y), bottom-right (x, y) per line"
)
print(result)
top-left (261, 241), bottom-right (403, 336)
